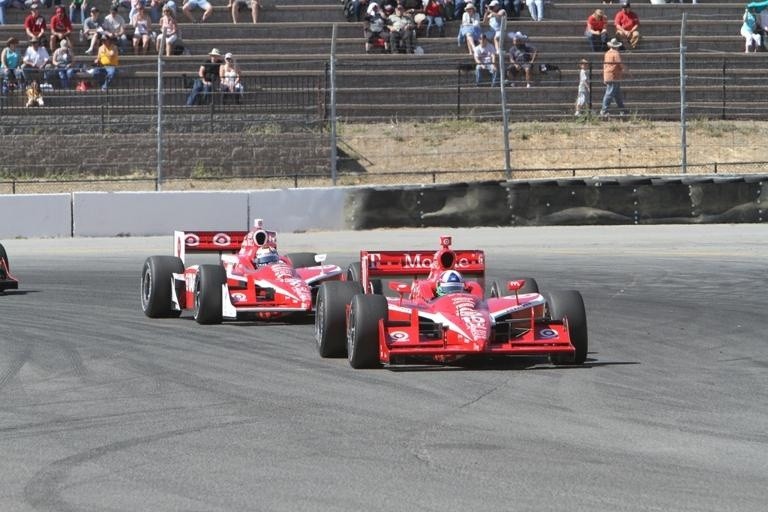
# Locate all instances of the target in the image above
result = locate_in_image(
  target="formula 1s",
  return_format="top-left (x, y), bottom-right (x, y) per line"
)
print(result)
top-left (137, 214), bottom-right (346, 327)
top-left (307, 233), bottom-right (590, 372)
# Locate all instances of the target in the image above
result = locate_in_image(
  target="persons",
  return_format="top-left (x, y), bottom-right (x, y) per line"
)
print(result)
top-left (574, 59), bottom-right (589, 116)
top-left (740, 7), bottom-right (762, 53)
top-left (758, 6), bottom-right (768, 52)
top-left (474, 33), bottom-right (498, 88)
top-left (599, 38), bottom-right (630, 116)
top-left (436, 270), bottom-right (469, 297)
top-left (255, 246), bottom-right (286, 269)
top-left (343, 0), bottom-right (546, 55)
top-left (504, 31), bottom-right (537, 89)
top-left (584, 10), bottom-right (608, 52)
top-left (0, 0), bottom-right (263, 107)
top-left (614, 2), bottom-right (640, 52)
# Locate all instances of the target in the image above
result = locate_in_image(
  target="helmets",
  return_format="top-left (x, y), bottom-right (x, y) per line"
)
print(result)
top-left (254, 245), bottom-right (279, 268)
top-left (437, 270), bottom-right (465, 294)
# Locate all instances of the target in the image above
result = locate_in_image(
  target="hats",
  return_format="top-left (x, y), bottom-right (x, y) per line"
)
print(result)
top-left (507, 31), bottom-right (528, 42)
top-left (6, 34), bottom-right (116, 50)
top-left (606, 37), bottom-right (623, 48)
top-left (208, 47), bottom-right (221, 56)
top-left (488, 0), bottom-right (500, 7)
top-left (463, 3), bottom-right (477, 11)
top-left (476, 33), bottom-right (488, 40)
top-left (90, 4), bottom-right (119, 14)
top-left (224, 52), bottom-right (233, 60)
top-left (620, 1), bottom-right (631, 8)
top-left (29, 4), bottom-right (66, 19)
